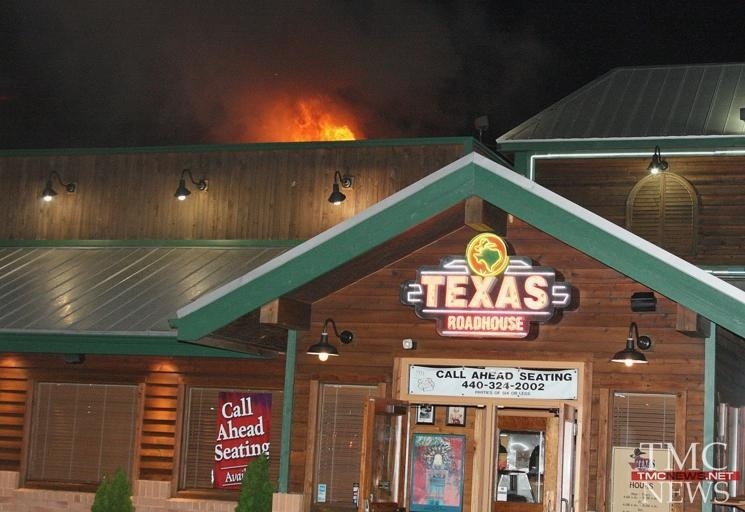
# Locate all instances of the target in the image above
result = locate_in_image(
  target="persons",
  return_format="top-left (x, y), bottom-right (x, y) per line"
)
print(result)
top-left (420, 406), bottom-right (431, 418)
top-left (450, 407), bottom-right (462, 424)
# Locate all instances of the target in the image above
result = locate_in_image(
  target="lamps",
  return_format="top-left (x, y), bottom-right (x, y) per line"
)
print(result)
top-left (329, 170), bottom-right (354, 207)
top-left (175, 169), bottom-right (208, 202)
top-left (39, 170), bottom-right (74, 204)
top-left (647, 143), bottom-right (669, 175)
top-left (611, 321), bottom-right (652, 368)
top-left (306, 318), bottom-right (356, 364)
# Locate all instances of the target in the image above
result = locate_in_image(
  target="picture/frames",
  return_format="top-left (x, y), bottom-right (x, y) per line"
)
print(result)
top-left (409, 431), bottom-right (466, 512)
top-left (414, 403), bottom-right (435, 425)
top-left (445, 406), bottom-right (466, 427)
